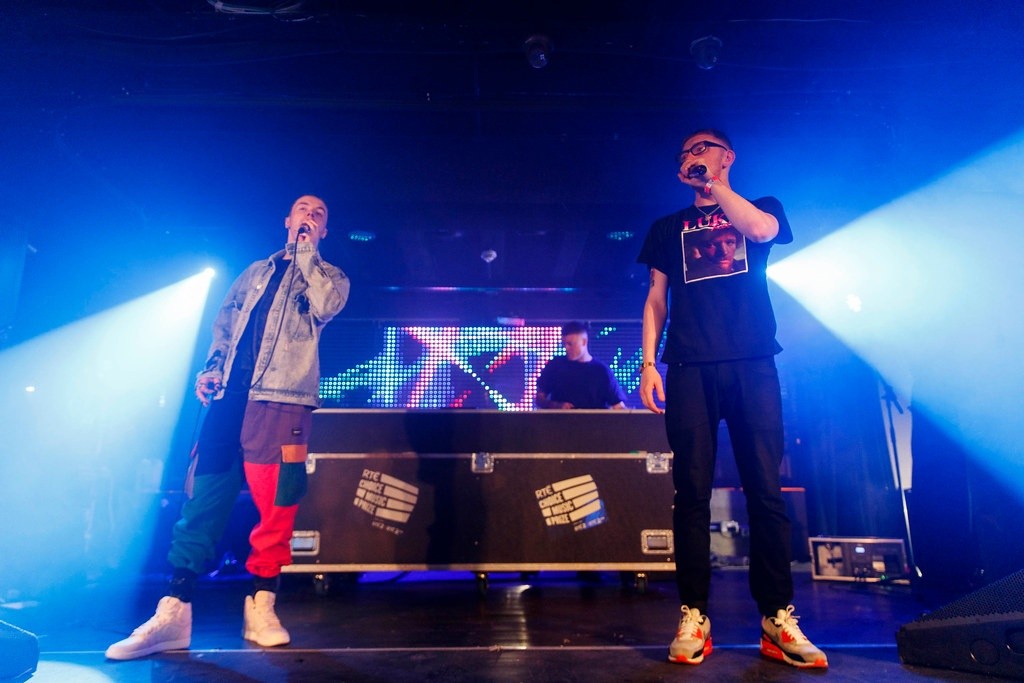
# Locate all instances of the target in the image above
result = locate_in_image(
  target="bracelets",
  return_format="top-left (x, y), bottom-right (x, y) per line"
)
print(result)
top-left (703, 175), bottom-right (720, 195)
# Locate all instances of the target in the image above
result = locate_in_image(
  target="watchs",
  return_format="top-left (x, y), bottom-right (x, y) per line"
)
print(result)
top-left (640, 361), bottom-right (657, 373)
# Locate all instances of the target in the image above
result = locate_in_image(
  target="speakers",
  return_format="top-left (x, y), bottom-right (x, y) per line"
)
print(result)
top-left (895, 570), bottom-right (1024, 683)
top-left (0, 621), bottom-right (38, 683)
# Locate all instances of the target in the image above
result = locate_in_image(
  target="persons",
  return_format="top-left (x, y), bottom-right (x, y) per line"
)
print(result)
top-left (639, 127), bottom-right (831, 668)
top-left (104, 194), bottom-right (350, 660)
top-left (535, 320), bottom-right (629, 409)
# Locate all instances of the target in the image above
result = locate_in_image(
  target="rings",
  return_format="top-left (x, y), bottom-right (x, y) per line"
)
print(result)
top-left (198, 383), bottom-right (207, 388)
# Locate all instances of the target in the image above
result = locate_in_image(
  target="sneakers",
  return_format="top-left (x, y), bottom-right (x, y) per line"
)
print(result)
top-left (760, 604), bottom-right (829, 668)
top-left (105, 596), bottom-right (192, 660)
top-left (241, 590), bottom-right (291, 647)
top-left (667, 604), bottom-right (714, 665)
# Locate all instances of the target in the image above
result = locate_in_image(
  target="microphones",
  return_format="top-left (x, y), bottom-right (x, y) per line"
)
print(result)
top-left (298, 223), bottom-right (310, 234)
top-left (687, 165), bottom-right (707, 178)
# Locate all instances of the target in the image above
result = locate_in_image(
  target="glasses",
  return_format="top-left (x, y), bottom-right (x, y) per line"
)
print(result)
top-left (675, 139), bottom-right (728, 165)
